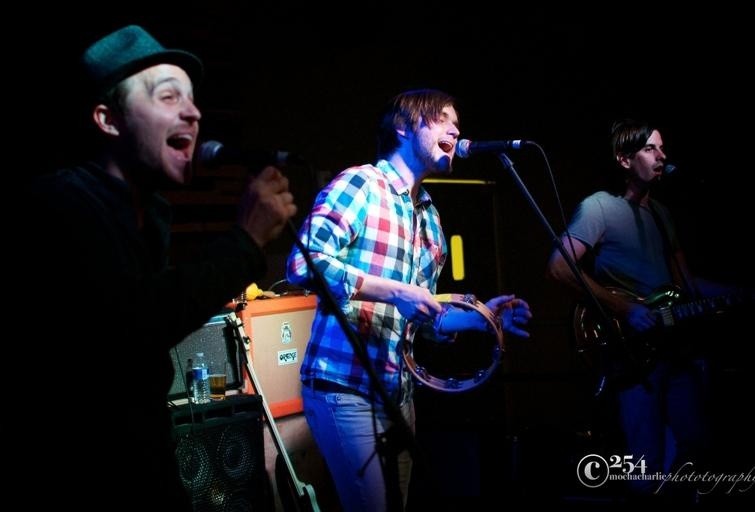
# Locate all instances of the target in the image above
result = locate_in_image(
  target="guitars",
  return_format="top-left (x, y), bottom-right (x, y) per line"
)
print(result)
top-left (574, 288), bottom-right (755, 392)
top-left (235, 318), bottom-right (321, 511)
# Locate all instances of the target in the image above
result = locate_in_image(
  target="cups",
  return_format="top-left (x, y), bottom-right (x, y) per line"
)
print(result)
top-left (209, 360), bottom-right (227, 402)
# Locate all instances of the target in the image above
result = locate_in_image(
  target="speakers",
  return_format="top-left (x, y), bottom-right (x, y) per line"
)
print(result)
top-left (170, 394), bottom-right (275, 512)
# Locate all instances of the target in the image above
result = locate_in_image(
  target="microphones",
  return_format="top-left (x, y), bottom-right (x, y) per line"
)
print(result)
top-left (665, 163), bottom-right (705, 188)
top-left (198, 140), bottom-right (261, 183)
top-left (455, 138), bottom-right (524, 159)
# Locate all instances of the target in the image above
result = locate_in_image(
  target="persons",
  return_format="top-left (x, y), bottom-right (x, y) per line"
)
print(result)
top-left (286, 88), bottom-right (532, 512)
top-left (547, 120), bottom-right (701, 489)
top-left (0, 26), bottom-right (298, 512)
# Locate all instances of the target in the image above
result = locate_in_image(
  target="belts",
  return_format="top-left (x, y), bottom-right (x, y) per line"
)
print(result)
top-left (301, 381), bottom-right (367, 398)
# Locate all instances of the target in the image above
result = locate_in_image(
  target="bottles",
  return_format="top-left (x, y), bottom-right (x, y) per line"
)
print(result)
top-left (192, 352), bottom-right (209, 405)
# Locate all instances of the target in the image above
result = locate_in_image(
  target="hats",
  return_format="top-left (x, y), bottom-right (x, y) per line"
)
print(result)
top-left (81, 25), bottom-right (203, 96)
